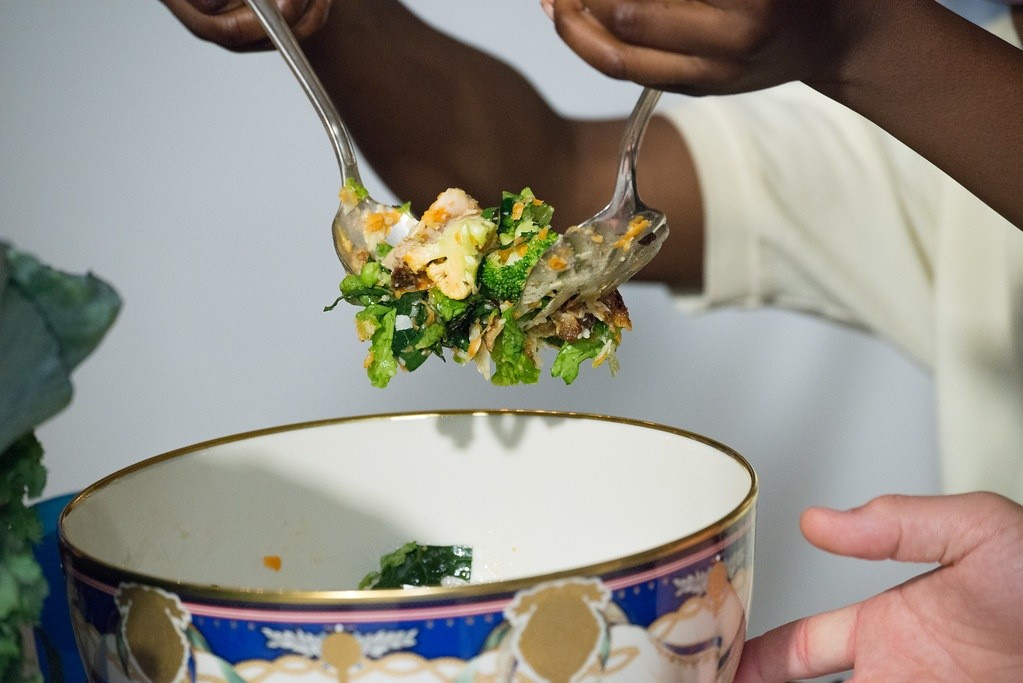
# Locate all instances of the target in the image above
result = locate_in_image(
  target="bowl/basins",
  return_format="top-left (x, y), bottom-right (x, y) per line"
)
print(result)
top-left (57, 411), bottom-right (758, 682)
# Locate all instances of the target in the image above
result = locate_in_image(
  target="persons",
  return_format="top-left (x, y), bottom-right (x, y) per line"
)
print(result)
top-left (158, 0), bottom-right (1022, 510)
top-left (735, 492), bottom-right (1023, 683)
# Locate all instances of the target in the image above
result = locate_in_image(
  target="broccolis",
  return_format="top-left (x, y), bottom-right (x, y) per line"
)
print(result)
top-left (385, 187), bottom-right (494, 300)
top-left (481, 231), bottom-right (565, 302)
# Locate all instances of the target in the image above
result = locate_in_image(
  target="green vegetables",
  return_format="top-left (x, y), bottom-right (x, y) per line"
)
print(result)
top-left (357, 542), bottom-right (474, 586)
top-left (330, 186), bottom-right (623, 383)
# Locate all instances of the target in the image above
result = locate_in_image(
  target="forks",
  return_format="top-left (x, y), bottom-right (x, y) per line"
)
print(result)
top-left (512, 87), bottom-right (670, 330)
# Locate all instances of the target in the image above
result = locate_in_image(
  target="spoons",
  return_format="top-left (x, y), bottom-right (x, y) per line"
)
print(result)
top-left (245, 2), bottom-right (419, 274)
